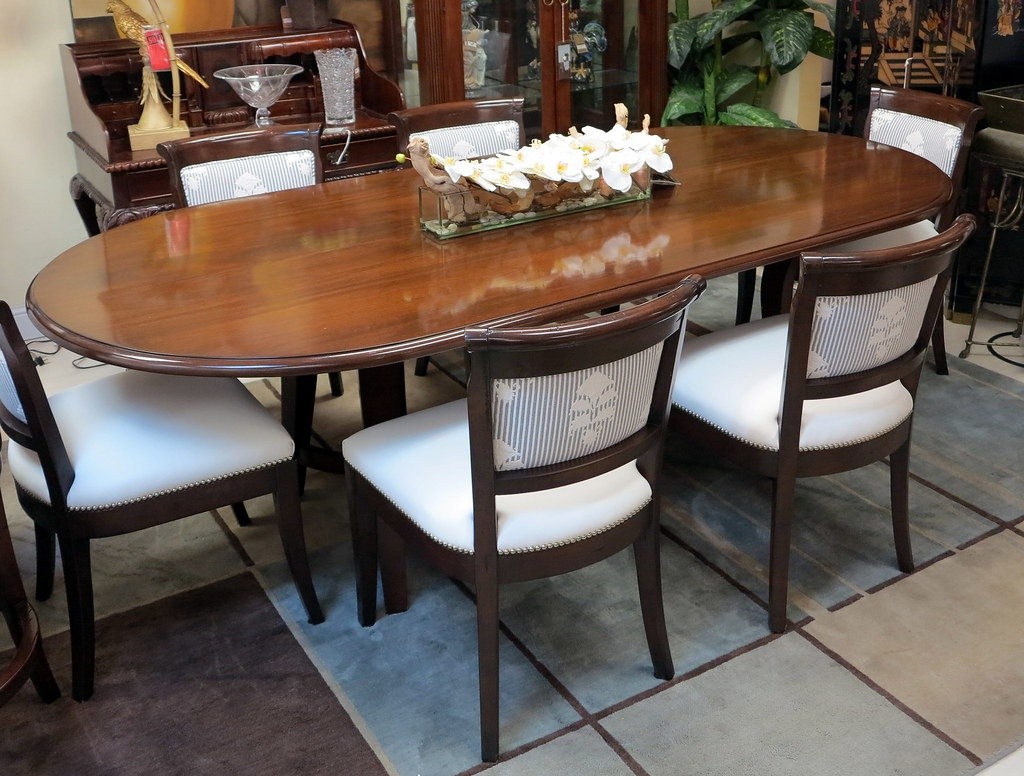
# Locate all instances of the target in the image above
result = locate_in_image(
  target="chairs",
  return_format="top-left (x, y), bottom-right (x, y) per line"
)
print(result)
top-left (733, 84), bottom-right (985, 374)
top-left (388, 95), bottom-right (528, 377)
top-left (156, 119), bottom-right (345, 399)
top-left (341, 272), bottom-right (708, 766)
top-left (0, 300), bottom-right (325, 703)
top-left (664, 212), bottom-right (979, 633)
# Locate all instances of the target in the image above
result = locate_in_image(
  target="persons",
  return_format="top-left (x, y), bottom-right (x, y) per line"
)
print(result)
top-left (461, 0), bottom-right (486, 88)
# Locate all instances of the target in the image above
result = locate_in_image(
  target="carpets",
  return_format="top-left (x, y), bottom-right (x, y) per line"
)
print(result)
top-left (201, 272), bottom-right (1024, 776)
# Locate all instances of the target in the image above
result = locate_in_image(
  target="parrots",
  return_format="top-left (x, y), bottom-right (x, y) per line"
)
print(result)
top-left (105, 0), bottom-right (210, 88)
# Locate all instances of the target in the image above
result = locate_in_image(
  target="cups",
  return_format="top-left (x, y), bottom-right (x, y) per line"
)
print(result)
top-left (313, 47), bottom-right (357, 130)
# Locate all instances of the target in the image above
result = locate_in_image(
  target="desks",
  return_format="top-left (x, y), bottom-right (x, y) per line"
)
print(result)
top-left (23, 124), bottom-right (954, 614)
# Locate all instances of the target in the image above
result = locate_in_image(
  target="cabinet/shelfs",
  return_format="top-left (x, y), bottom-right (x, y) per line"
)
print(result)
top-left (386, 0), bottom-right (669, 140)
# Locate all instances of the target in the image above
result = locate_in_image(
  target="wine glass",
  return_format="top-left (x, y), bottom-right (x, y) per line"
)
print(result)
top-left (213, 63), bottom-right (304, 131)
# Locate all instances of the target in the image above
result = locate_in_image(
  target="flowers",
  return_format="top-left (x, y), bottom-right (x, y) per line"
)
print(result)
top-left (437, 100), bottom-right (677, 194)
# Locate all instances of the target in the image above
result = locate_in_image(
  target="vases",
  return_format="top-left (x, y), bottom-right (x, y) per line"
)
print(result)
top-left (417, 162), bottom-right (652, 239)
